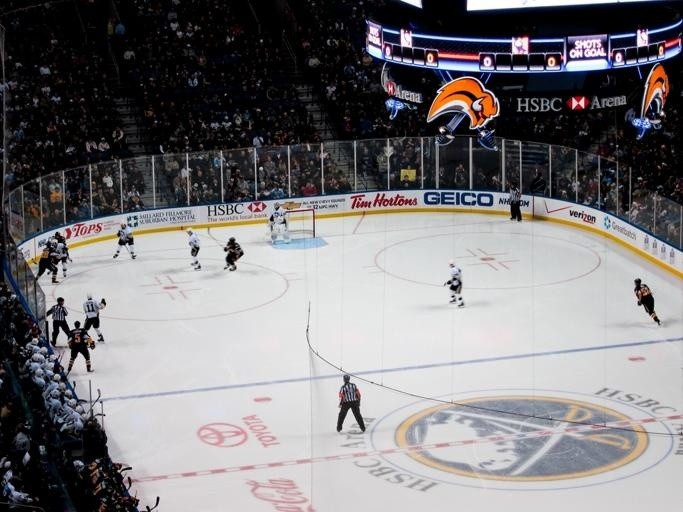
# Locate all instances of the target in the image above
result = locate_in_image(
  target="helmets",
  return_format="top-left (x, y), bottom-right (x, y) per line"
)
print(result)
top-left (26, 336), bottom-right (84, 421)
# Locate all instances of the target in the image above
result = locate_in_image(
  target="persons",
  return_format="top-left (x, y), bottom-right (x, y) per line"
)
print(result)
top-left (34, 240), bottom-right (59, 284)
top-left (45, 238), bottom-right (73, 278)
top-left (0, 276), bottom-right (133, 512)
top-left (631, 276), bottom-right (662, 326)
top-left (221, 236), bottom-right (244, 271)
top-left (0, 1), bottom-right (682, 250)
top-left (185, 229), bottom-right (201, 271)
top-left (111, 222), bottom-right (137, 260)
top-left (331, 373), bottom-right (369, 434)
top-left (441, 260), bottom-right (465, 308)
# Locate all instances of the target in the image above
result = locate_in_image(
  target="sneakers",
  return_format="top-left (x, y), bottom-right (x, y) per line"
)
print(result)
top-left (130, 255), bottom-right (137, 260)
top-left (51, 280), bottom-right (60, 283)
top-left (97, 337), bottom-right (105, 341)
top-left (113, 254), bottom-right (118, 258)
top-left (190, 263), bottom-right (201, 270)
top-left (224, 263), bottom-right (237, 271)
top-left (449, 299), bottom-right (455, 303)
top-left (458, 303), bottom-right (464, 307)
